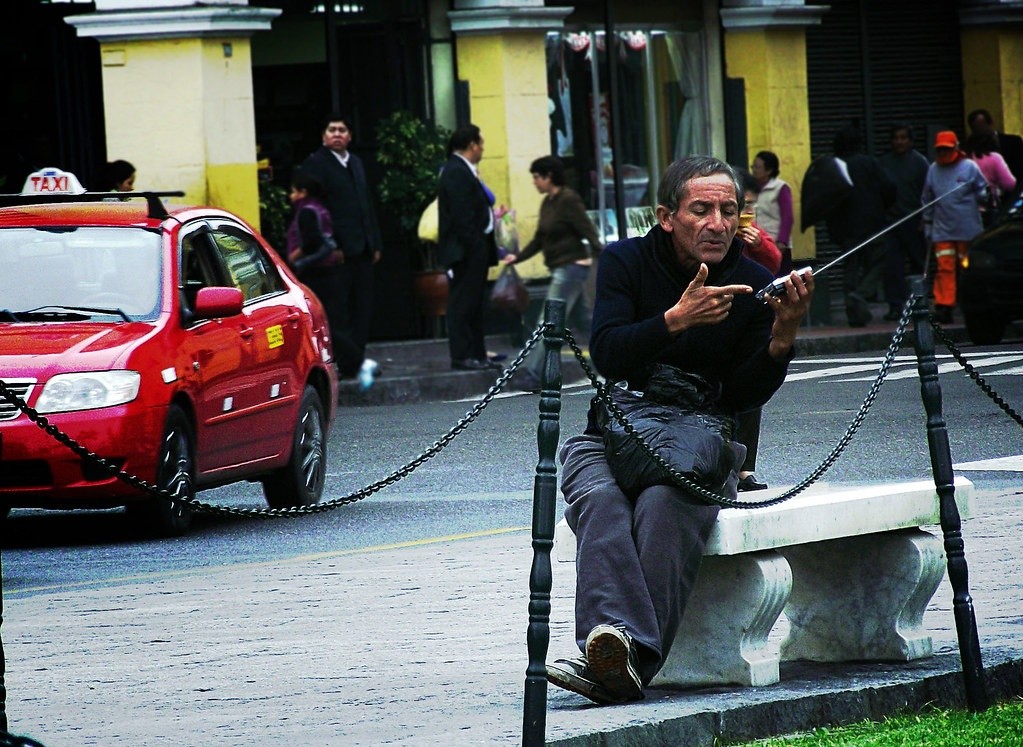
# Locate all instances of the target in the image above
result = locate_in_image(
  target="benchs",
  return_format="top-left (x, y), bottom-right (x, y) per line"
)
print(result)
top-left (647, 476), bottom-right (977, 687)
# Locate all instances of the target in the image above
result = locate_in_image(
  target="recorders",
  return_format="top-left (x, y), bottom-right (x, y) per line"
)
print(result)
top-left (755, 177), bottom-right (976, 305)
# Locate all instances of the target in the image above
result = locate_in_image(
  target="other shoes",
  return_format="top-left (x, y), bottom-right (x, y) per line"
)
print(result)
top-left (487, 352), bottom-right (507, 360)
top-left (513, 378), bottom-right (542, 389)
top-left (738, 475), bottom-right (767, 490)
top-left (355, 358), bottom-right (379, 393)
top-left (451, 357), bottom-right (502, 370)
top-left (934, 306), bottom-right (954, 324)
top-left (883, 304), bottom-right (902, 319)
top-left (339, 368), bottom-right (381, 378)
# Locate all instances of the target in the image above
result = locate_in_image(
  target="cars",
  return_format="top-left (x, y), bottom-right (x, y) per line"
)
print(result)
top-left (955, 176), bottom-right (1023, 343)
top-left (0, 168), bottom-right (338, 537)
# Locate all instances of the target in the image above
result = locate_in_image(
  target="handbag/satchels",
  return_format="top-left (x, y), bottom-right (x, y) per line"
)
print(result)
top-left (416, 199), bottom-right (440, 244)
top-left (292, 205), bottom-right (340, 279)
top-left (488, 264), bottom-right (530, 314)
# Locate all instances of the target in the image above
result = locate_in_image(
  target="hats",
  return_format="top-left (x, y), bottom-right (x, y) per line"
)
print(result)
top-left (933, 130), bottom-right (958, 148)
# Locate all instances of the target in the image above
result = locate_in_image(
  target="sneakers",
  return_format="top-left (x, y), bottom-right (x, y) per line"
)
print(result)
top-left (585, 624), bottom-right (643, 699)
top-left (546, 656), bottom-right (621, 705)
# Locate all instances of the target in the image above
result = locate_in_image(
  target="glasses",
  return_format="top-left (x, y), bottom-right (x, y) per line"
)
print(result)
top-left (937, 148), bottom-right (952, 152)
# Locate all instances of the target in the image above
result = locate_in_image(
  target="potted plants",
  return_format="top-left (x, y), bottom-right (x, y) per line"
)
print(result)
top-left (371, 111), bottom-right (452, 316)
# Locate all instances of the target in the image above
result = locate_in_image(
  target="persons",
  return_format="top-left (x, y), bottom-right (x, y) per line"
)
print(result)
top-left (437, 125), bottom-right (510, 369)
top-left (287, 116), bottom-right (382, 379)
top-left (545, 157), bottom-right (815, 705)
top-left (751, 151), bottom-right (794, 278)
top-left (504, 156), bottom-right (603, 395)
top-left (827, 109), bottom-right (1023, 328)
top-left (102, 161), bottom-right (136, 202)
